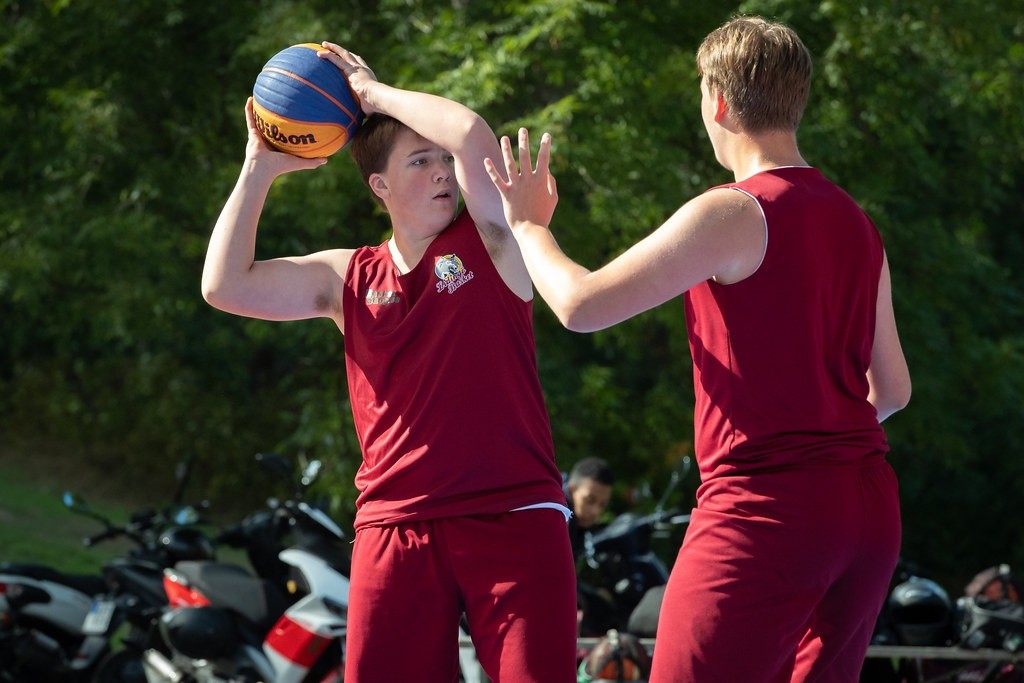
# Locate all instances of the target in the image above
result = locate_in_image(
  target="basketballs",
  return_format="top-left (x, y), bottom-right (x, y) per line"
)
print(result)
top-left (251, 43), bottom-right (363, 159)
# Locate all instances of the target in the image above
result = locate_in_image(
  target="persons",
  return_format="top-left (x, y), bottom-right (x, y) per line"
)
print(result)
top-left (567, 457), bottom-right (614, 619)
top-left (201, 41), bottom-right (577, 683)
top-left (484, 14), bottom-right (912, 683)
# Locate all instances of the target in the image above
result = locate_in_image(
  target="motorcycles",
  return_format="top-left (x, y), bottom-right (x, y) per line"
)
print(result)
top-left (0, 454), bottom-right (1023, 683)
